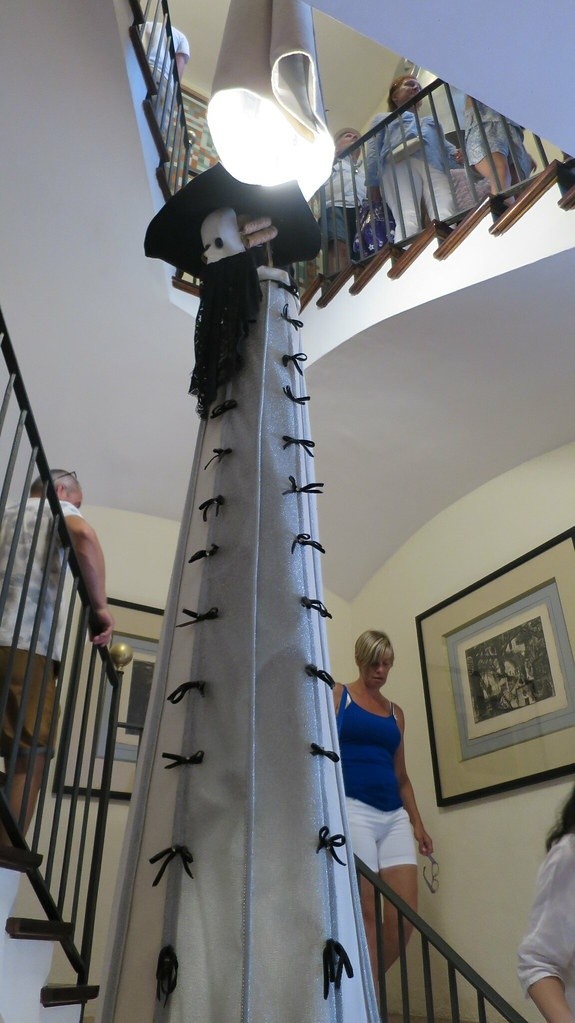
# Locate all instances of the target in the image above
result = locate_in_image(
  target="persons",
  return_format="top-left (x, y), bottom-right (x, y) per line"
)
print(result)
top-left (0, 469), bottom-right (115, 847)
top-left (515, 783), bottom-right (575, 1023)
top-left (138, 21), bottom-right (190, 139)
top-left (332, 630), bottom-right (433, 1003)
top-left (317, 76), bottom-right (537, 275)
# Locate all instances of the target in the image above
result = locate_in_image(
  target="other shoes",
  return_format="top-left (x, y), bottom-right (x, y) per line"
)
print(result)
top-left (0, 845), bottom-right (44, 872)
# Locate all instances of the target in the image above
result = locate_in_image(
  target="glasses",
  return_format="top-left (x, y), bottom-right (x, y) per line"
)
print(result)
top-left (422, 855), bottom-right (439, 894)
top-left (53, 471), bottom-right (77, 481)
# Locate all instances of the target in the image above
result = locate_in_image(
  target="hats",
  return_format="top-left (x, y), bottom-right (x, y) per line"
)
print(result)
top-left (334, 127), bottom-right (362, 142)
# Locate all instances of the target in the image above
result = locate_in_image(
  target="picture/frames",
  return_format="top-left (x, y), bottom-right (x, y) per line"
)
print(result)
top-left (52, 598), bottom-right (164, 800)
top-left (163, 83), bottom-right (222, 196)
top-left (416, 526), bottom-right (575, 807)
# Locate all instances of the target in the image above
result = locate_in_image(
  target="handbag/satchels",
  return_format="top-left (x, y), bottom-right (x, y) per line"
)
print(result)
top-left (352, 198), bottom-right (396, 257)
